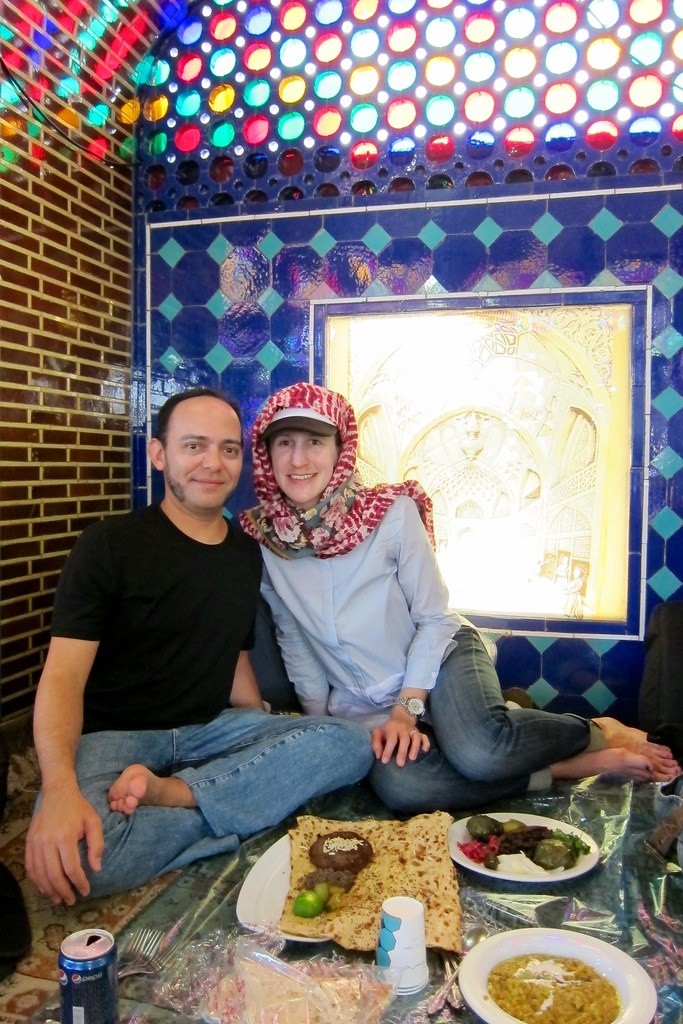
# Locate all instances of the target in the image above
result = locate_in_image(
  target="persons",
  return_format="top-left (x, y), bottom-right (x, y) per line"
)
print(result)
top-left (24, 390), bottom-right (375, 906)
top-left (238, 382), bottom-right (682, 814)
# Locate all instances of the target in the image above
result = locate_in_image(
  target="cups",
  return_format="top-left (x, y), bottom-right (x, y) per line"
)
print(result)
top-left (372, 896), bottom-right (430, 996)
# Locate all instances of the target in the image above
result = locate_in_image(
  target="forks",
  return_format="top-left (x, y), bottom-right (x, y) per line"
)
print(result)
top-left (45, 928), bottom-right (181, 1010)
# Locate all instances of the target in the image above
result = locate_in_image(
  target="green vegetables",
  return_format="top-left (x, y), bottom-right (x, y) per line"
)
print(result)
top-left (553, 829), bottom-right (591, 856)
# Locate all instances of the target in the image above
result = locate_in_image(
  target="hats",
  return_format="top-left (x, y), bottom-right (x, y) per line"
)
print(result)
top-left (259, 403), bottom-right (338, 441)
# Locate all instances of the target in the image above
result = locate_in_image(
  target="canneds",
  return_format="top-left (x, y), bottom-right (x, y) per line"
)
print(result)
top-left (60, 928), bottom-right (117, 1024)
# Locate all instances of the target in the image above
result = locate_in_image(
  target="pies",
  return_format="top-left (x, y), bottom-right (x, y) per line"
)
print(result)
top-left (275, 809), bottom-right (462, 953)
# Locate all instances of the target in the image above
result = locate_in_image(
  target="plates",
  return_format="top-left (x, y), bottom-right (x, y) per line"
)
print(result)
top-left (237, 832), bottom-right (329, 944)
top-left (447, 812), bottom-right (600, 883)
top-left (458, 928), bottom-right (657, 1024)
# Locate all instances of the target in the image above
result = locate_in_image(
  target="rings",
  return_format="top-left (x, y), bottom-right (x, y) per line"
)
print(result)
top-left (409, 730), bottom-right (416, 737)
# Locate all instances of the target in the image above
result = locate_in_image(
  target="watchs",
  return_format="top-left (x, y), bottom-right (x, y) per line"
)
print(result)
top-left (393, 697), bottom-right (426, 720)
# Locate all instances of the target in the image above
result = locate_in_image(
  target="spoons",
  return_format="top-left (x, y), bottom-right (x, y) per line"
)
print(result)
top-left (426, 928), bottom-right (489, 1015)
